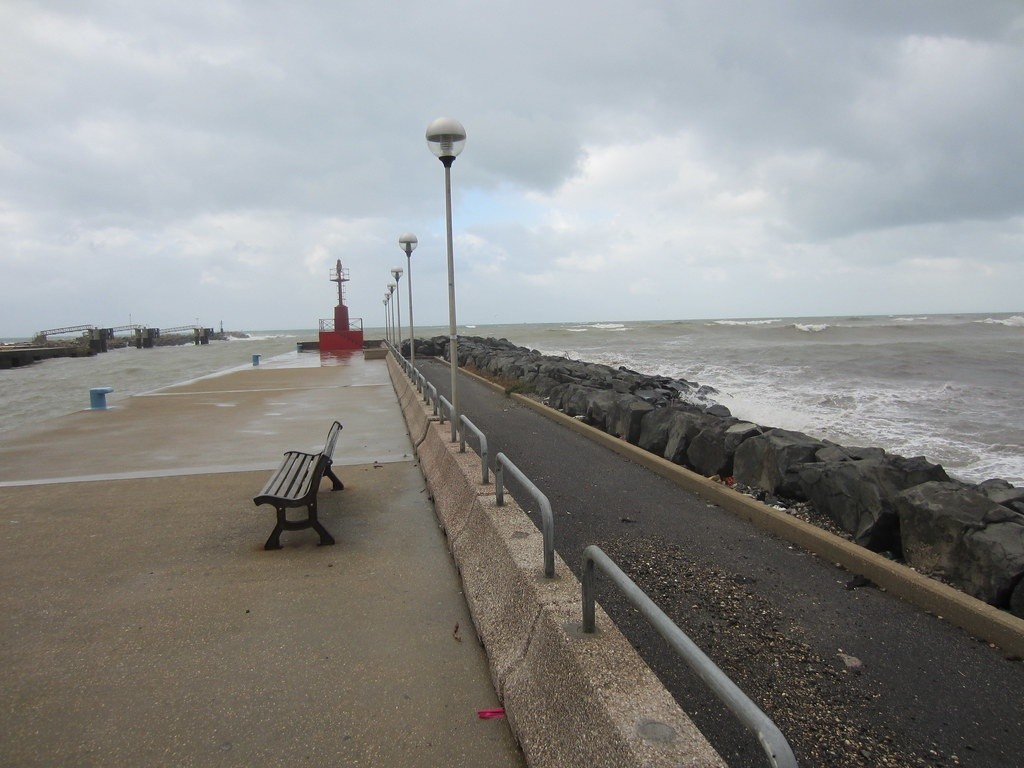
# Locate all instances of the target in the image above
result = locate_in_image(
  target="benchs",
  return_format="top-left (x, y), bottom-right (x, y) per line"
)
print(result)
top-left (252, 420), bottom-right (344, 551)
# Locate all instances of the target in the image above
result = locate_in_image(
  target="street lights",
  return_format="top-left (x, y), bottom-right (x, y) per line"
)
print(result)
top-left (423, 117), bottom-right (468, 444)
top-left (384, 291), bottom-right (391, 344)
top-left (387, 281), bottom-right (397, 348)
top-left (382, 299), bottom-right (387, 339)
top-left (390, 266), bottom-right (403, 355)
top-left (397, 232), bottom-right (417, 384)
